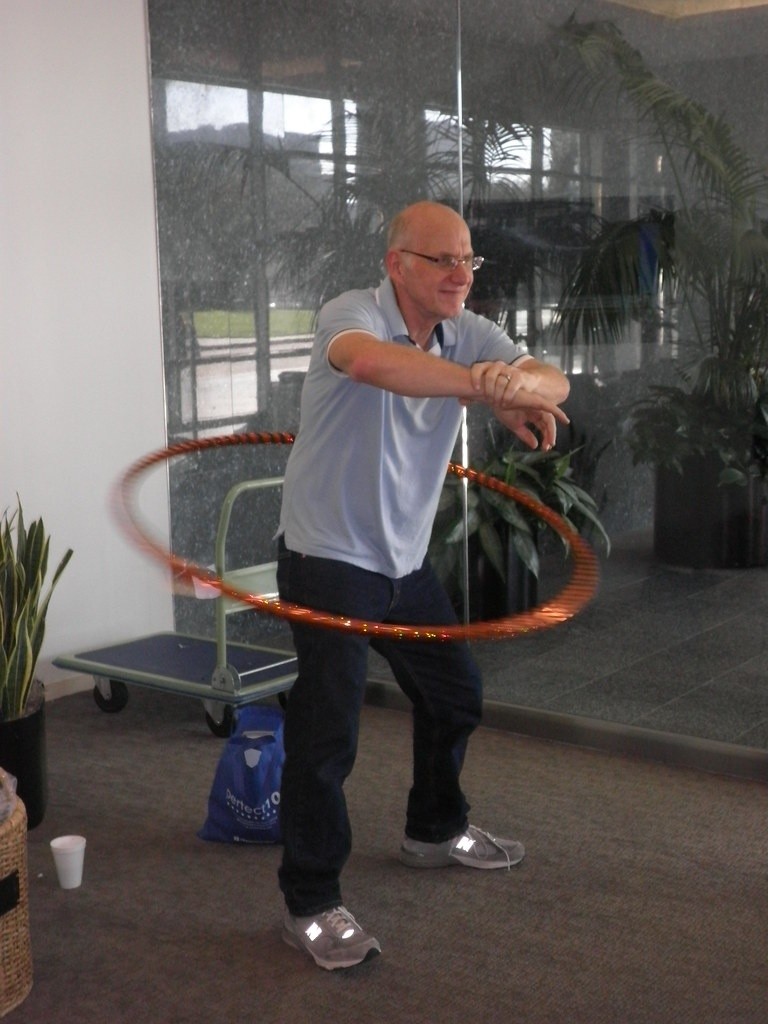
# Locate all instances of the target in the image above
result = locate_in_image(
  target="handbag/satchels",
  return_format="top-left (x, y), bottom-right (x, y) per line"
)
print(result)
top-left (197, 705), bottom-right (284, 845)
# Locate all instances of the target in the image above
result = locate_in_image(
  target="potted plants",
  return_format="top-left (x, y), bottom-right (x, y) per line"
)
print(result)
top-left (426, 420), bottom-right (612, 629)
top-left (530, 7), bottom-right (768, 575)
top-left (0, 489), bottom-right (74, 832)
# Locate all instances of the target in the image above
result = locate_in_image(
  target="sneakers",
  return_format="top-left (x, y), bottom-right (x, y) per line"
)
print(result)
top-left (283, 906), bottom-right (381, 970)
top-left (398, 825), bottom-right (527, 871)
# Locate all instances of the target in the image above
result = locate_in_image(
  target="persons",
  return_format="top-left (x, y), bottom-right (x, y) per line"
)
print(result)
top-left (272, 202), bottom-right (570, 971)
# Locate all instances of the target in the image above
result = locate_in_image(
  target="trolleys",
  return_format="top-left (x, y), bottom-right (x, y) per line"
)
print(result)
top-left (50, 475), bottom-right (301, 738)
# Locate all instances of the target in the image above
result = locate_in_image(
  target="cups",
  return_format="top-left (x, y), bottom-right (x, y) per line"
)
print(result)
top-left (50, 835), bottom-right (86, 889)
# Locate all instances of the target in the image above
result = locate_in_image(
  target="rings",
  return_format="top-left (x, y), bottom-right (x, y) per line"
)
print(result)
top-left (498, 374), bottom-right (510, 381)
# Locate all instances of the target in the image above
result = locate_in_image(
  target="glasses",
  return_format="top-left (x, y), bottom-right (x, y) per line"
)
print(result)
top-left (401, 249), bottom-right (485, 271)
